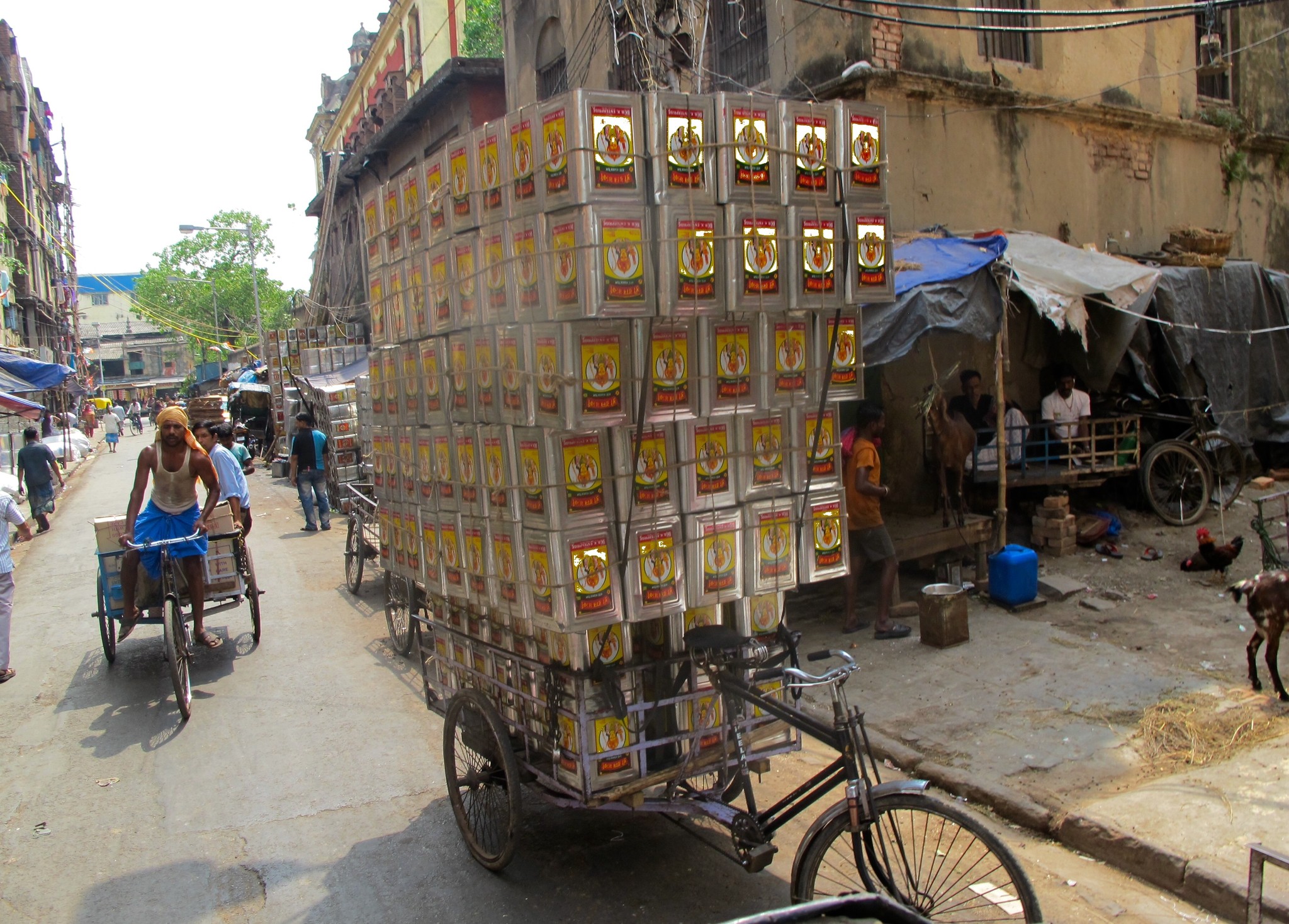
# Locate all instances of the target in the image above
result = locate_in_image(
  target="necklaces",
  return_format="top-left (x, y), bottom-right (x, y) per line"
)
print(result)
top-left (1064, 389), bottom-right (1073, 412)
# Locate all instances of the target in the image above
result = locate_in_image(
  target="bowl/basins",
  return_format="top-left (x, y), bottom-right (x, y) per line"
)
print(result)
top-left (921, 583), bottom-right (963, 601)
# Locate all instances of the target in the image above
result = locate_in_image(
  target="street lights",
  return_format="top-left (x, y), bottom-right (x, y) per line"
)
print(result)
top-left (178, 222), bottom-right (265, 367)
top-left (91, 322), bottom-right (106, 398)
top-left (166, 275), bottom-right (224, 378)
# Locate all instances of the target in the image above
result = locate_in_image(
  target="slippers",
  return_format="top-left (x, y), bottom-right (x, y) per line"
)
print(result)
top-left (843, 619), bottom-right (868, 633)
top-left (1096, 543), bottom-right (1122, 558)
top-left (197, 631), bottom-right (224, 649)
top-left (1140, 547), bottom-right (1163, 560)
top-left (117, 612), bottom-right (145, 642)
top-left (874, 623), bottom-right (912, 639)
top-left (0, 668), bottom-right (16, 681)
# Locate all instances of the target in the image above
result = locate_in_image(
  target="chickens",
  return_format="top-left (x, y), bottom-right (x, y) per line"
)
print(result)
top-left (1196, 527), bottom-right (1244, 585)
top-left (1179, 551), bottom-right (1214, 571)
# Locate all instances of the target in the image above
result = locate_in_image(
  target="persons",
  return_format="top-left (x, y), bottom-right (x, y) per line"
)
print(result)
top-left (0, 488), bottom-right (33, 684)
top-left (113, 402), bottom-right (125, 437)
top-left (950, 369), bottom-right (1030, 472)
top-left (39, 400), bottom-right (97, 438)
top-left (841, 401), bottom-right (913, 640)
top-left (1041, 370), bottom-right (1100, 465)
top-left (192, 419), bottom-right (252, 538)
top-left (145, 392), bottom-right (184, 430)
top-left (117, 406), bottom-right (223, 649)
top-left (102, 405), bottom-right (121, 453)
top-left (217, 423), bottom-right (255, 475)
top-left (17, 427), bottom-right (65, 533)
top-left (126, 398), bottom-right (143, 432)
top-left (290, 412), bottom-right (331, 531)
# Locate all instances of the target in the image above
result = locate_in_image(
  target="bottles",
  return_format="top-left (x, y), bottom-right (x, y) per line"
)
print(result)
top-left (1117, 431), bottom-right (1135, 465)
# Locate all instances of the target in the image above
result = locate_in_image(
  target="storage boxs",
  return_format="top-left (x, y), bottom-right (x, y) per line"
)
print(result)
top-left (0, 390), bottom-right (59, 485)
top-left (264, 88), bottom-right (896, 795)
top-left (93, 500), bottom-right (241, 610)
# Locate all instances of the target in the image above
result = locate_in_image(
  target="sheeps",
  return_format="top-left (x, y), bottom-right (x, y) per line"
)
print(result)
top-left (1225, 570), bottom-right (1289, 702)
top-left (922, 384), bottom-right (976, 527)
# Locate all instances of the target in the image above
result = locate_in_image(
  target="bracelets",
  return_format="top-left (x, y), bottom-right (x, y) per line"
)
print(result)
top-left (884, 487), bottom-right (888, 497)
top-left (1085, 446), bottom-right (1090, 449)
top-left (1072, 444), bottom-right (1076, 451)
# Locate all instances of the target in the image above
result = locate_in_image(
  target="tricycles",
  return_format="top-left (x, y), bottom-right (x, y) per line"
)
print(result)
top-left (922, 392), bottom-right (1248, 527)
top-left (344, 482), bottom-right (428, 655)
top-left (90, 527), bottom-right (265, 721)
top-left (421, 611), bottom-right (1047, 923)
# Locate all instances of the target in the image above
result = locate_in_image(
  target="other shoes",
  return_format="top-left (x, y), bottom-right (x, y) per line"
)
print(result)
top-left (300, 527), bottom-right (313, 530)
top-left (39, 513), bottom-right (50, 530)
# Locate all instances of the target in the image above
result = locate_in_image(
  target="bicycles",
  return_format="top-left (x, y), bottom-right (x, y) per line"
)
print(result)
top-left (146, 407), bottom-right (158, 427)
top-left (127, 411), bottom-right (143, 436)
